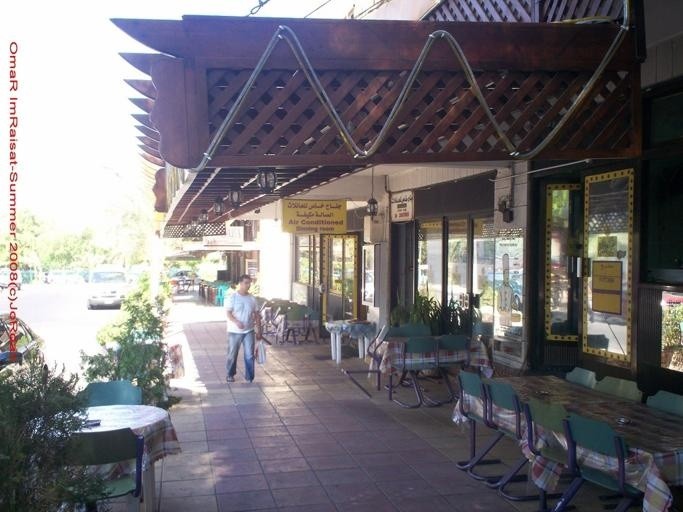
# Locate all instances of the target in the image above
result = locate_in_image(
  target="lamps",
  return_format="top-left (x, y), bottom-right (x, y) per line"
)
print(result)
top-left (366, 164), bottom-right (378, 216)
top-left (255, 167), bottom-right (276, 194)
top-left (191, 185), bottom-right (242, 228)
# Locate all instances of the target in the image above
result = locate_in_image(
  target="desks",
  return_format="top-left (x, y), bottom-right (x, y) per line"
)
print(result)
top-left (23, 405), bottom-right (171, 512)
top-left (325, 319), bottom-right (370, 364)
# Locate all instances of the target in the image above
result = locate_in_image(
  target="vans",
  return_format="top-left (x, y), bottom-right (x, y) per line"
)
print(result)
top-left (450, 238), bottom-right (521, 286)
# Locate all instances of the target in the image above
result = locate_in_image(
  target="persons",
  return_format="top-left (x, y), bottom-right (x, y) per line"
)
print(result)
top-left (222, 274), bottom-right (264, 386)
top-left (494, 253), bottom-right (518, 329)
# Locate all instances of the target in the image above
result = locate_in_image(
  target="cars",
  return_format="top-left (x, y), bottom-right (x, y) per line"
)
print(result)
top-left (0, 265), bottom-right (131, 311)
top-left (0, 313), bottom-right (45, 381)
top-left (496, 271), bottom-right (522, 312)
top-left (166, 267), bottom-right (203, 285)
top-left (337, 267), bottom-right (373, 300)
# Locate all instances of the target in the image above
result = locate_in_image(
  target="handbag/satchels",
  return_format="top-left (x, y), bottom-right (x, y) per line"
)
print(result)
top-left (254, 339), bottom-right (266, 363)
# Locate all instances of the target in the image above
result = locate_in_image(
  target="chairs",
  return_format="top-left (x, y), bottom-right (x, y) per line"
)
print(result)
top-left (340, 320), bottom-right (494, 409)
top-left (456, 366), bottom-right (682, 512)
top-left (253, 298), bottom-right (319, 346)
top-left (52, 379), bottom-right (145, 512)
